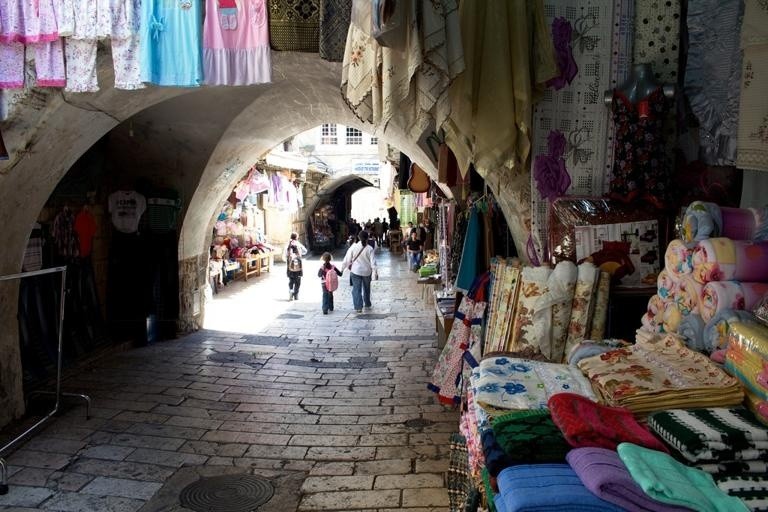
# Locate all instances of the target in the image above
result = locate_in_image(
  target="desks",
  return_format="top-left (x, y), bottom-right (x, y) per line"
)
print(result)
top-left (433, 290), bottom-right (457, 304)
top-left (253, 253), bottom-right (270, 276)
top-left (234, 257), bottom-right (258, 282)
top-left (417, 277), bottom-right (441, 303)
top-left (434, 306), bottom-right (457, 349)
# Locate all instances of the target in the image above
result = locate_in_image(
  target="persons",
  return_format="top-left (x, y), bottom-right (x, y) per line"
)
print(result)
top-left (341, 230), bottom-right (378, 313)
top-left (286, 245), bottom-right (303, 302)
top-left (401, 221), bottom-right (413, 253)
top-left (406, 232), bottom-right (423, 273)
top-left (343, 217), bottom-right (389, 246)
top-left (317, 252), bottom-right (343, 315)
top-left (281, 232), bottom-right (308, 262)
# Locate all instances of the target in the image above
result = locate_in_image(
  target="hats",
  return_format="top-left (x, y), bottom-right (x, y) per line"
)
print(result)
top-left (359, 231), bottom-right (369, 241)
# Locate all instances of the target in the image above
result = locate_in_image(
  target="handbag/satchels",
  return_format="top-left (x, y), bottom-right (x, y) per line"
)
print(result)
top-left (350, 246), bottom-right (365, 286)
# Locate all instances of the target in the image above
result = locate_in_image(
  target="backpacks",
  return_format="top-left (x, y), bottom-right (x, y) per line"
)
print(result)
top-left (320, 265), bottom-right (339, 292)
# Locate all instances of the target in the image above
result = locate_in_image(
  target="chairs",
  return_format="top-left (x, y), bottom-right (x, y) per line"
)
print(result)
top-left (407, 251), bottom-right (422, 271)
